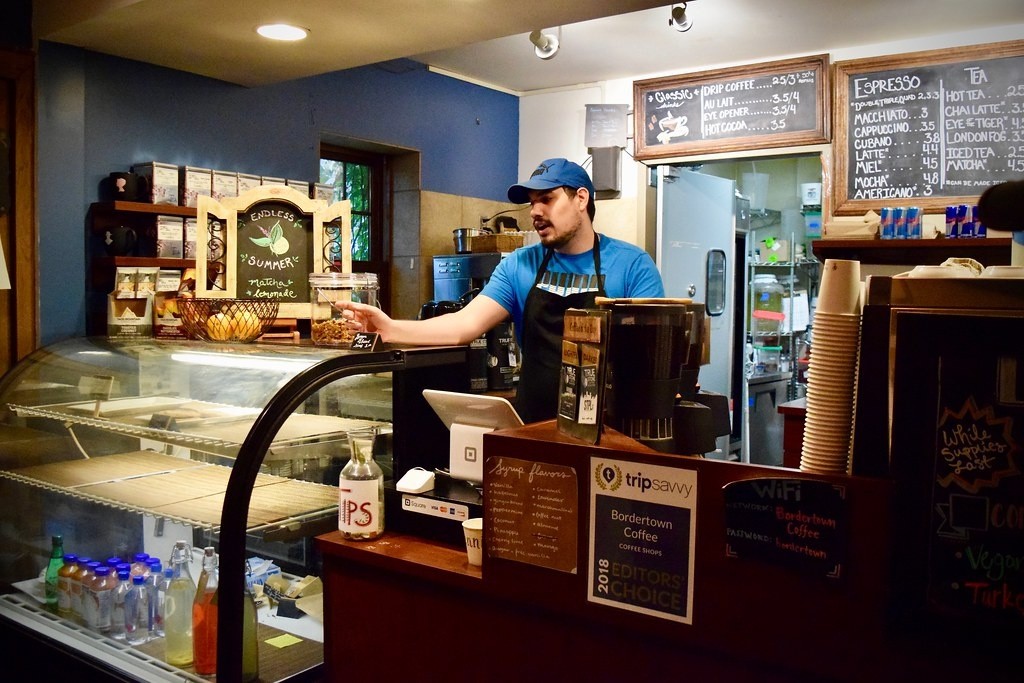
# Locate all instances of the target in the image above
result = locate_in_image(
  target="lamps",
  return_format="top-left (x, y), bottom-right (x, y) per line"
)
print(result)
top-left (529, 25), bottom-right (562, 60)
top-left (668, 2), bottom-right (693, 32)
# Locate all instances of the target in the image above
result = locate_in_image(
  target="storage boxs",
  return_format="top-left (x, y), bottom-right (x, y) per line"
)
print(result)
top-left (801, 182), bottom-right (823, 237)
top-left (780, 288), bottom-right (811, 332)
top-left (760, 240), bottom-right (790, 261)
top-left (107, 160), bottom-right (335, 335)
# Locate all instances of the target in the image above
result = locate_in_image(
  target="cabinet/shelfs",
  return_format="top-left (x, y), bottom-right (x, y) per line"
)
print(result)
top-left (91, 201), bottom-right (341, 270)
top-left (432, 250), bottom-right (513, 303)
top-left (747, 232), bottom-right (821, 467)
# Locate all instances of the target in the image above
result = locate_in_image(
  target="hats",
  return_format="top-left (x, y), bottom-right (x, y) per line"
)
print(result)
top-left (507, 158), bottom-right (594, 204)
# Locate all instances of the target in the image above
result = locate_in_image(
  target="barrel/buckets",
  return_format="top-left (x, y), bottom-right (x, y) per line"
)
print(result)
top-left (800, 182), bottom-right (821, 205)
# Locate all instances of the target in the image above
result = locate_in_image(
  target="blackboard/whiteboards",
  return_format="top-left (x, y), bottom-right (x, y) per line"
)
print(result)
top-left (832, 39), bottom-right (1024, 217)
top-left (237, 199), bottom-right (314, 303)
top-left (632, 53), bottom-right (833, 160)
top-left (921, 392), bottom-right (1024, 624)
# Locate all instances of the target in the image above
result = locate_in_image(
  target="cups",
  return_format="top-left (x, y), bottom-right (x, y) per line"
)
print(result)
top-left (462, 517), bottom-right (482, 566)
top-left (742, 172), bottom-right (769, 210)
top-left (800, 259), bottom-right (866, 477)
top-left (308, 272), bottom-right (381, 348)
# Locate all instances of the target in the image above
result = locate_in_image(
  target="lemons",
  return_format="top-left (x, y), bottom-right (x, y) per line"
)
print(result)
top-left (205, 312), bottom-right (261, 340)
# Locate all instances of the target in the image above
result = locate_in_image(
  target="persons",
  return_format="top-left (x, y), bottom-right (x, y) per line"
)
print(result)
top-left (335, 158), bottom-right (665, 426)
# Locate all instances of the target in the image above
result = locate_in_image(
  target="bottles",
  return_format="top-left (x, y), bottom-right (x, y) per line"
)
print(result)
top-left (742, 272), bottom-right (809, 377)
top-left (338, 431), bottom-right (385, 541)
top-left (43, 532), bottom-right (260, 683)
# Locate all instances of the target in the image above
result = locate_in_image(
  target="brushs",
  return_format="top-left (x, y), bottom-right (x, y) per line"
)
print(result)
top-left (595, 296), bottom-right (693, 305)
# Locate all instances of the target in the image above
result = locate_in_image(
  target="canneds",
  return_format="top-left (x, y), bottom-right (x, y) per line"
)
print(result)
top-left (879, 206), bottom-right (922, 239)
top-left (945, 205), bottom-right (986, 238)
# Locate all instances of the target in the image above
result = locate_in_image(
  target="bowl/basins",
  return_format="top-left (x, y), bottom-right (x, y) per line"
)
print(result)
top-left (452, 228), bottom-right (489, 254)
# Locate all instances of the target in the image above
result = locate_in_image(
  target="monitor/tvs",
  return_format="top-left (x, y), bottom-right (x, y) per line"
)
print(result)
top-left (422, 389), bottom-right (525, 484)
